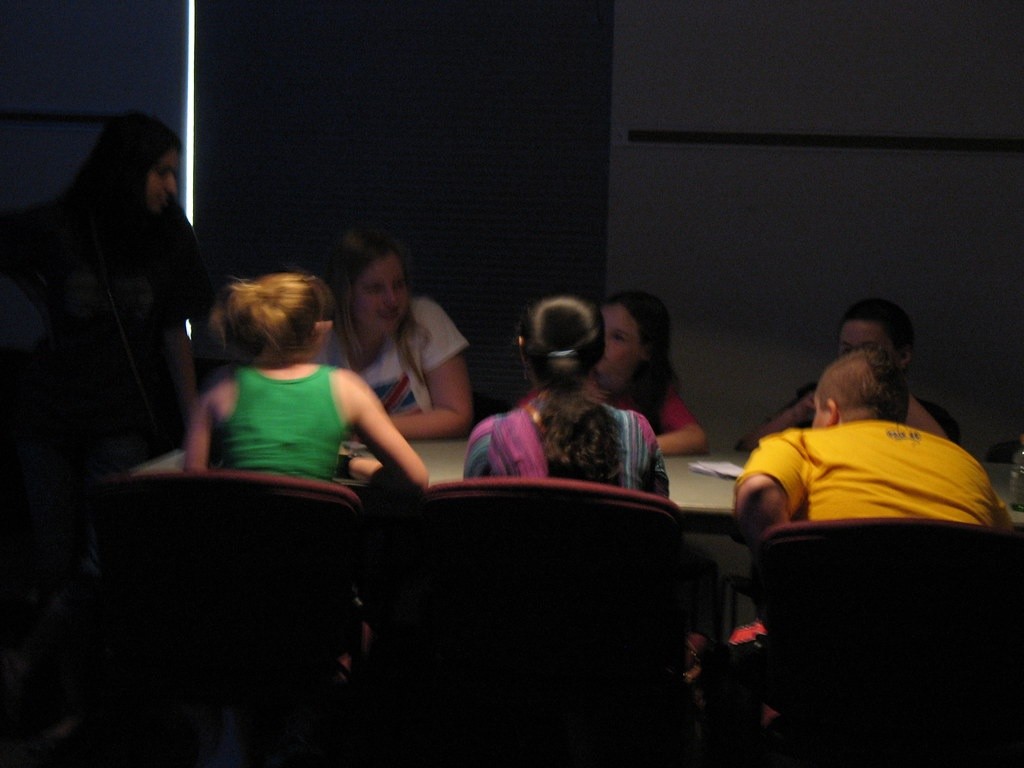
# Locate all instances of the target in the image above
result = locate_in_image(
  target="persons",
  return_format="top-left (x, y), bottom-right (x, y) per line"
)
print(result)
top-left (316, 226), bottom-right (475, 438)
top-left (181, 270), bottom-right (430, 494)
top-left (519, 284), bottom-right (709, 456)
top-left (0, 109), bottom-right (212, 725)
top-left (733, 348), bottom-right (1014, 559)
top-left (733, 297), bottom-right (961, 452)
top-left (456, 290), bottom-right (669, 502)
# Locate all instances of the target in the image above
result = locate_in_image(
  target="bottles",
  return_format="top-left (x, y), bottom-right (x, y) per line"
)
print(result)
top-left (1009, 435), bottom-right (1024, 512)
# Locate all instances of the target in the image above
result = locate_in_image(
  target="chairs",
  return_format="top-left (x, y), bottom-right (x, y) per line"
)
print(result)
top-left (90, 466), bottom-right (367, 768)
top-left (371, 476), bottom-right (713, 768)
top-left (735, 518), bottom-right (1024, 768)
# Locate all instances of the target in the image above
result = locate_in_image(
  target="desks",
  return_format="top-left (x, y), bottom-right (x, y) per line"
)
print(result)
top-left (137, 440), bottom-right (1024, 641)
